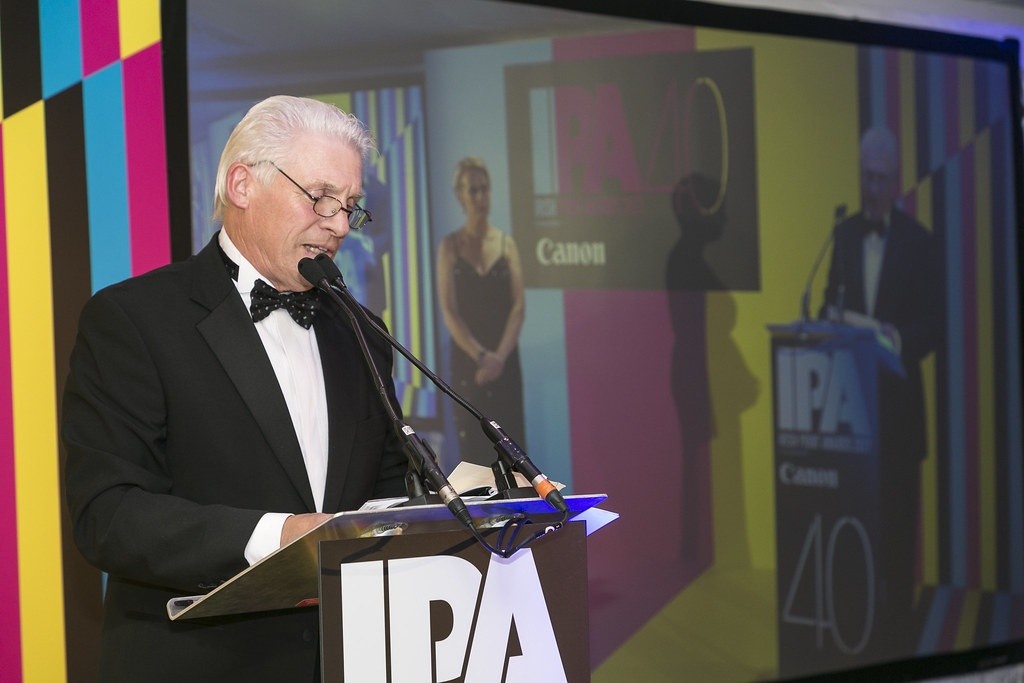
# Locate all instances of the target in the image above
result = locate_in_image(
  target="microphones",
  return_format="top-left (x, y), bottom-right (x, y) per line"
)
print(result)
top-left (314, 254), bottom-right (569, 515)
top-left (297, 257), bottom-right (472, 527)
top-left (801, 204), bottom-right (846, 322)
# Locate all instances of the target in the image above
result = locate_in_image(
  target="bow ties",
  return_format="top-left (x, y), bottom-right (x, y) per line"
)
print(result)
top-left (218, 245), bottom-right (322, 330)
top-left (862, 219), bottom-right (885, 239)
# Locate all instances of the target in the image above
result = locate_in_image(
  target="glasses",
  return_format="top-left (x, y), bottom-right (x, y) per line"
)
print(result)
top-left (246, 159), bottom-right (372, 230)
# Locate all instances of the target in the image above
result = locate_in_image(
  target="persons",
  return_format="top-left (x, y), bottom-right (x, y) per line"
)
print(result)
top-left (437, 156), bottom-right (526, 471)
top-left (811, 125), bottom-right (940, 612)
top-left (59, 94), bottom-right (412, 683)
